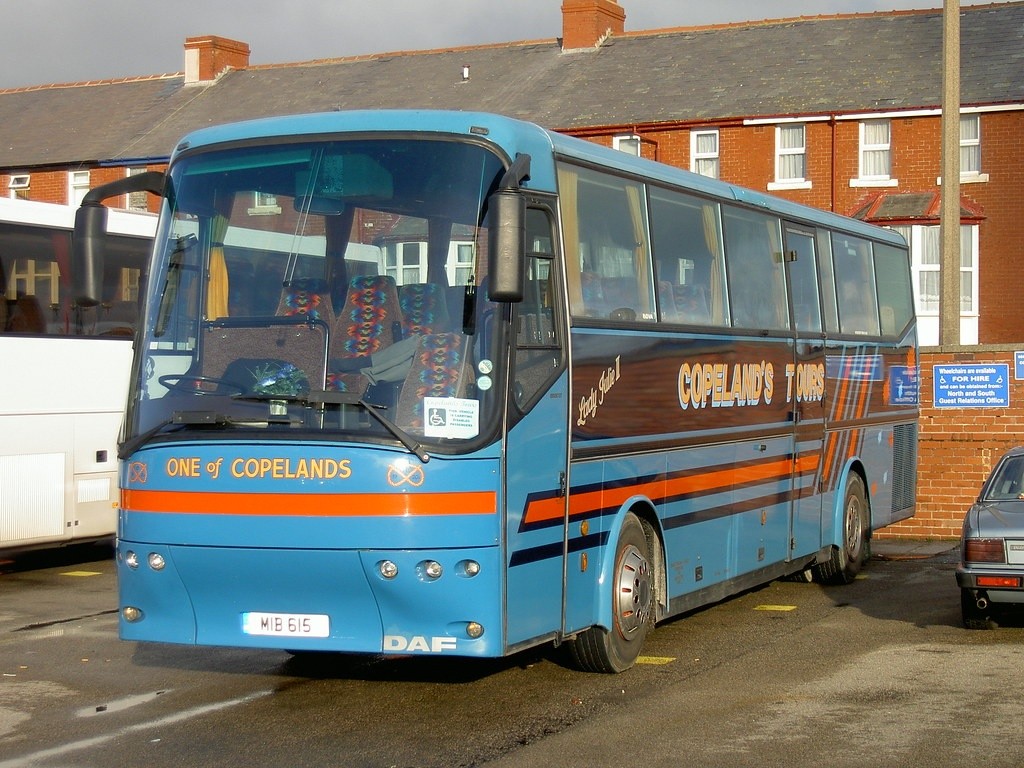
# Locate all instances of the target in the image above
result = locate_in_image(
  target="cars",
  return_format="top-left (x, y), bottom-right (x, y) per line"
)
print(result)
top-left (952, 445), bottom-right (1023, 630)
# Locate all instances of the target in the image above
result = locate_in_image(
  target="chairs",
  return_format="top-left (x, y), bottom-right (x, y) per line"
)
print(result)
top-left (395, 336), bottom-right (474, 436)
top-left (450, 271), bottom-right (816, 332)
top-left (0, 294), bottom-right (49, 335)
top-left (268, 276), bottom-right (449, 429)
top-left (108, 300), bottom-right (138, 335)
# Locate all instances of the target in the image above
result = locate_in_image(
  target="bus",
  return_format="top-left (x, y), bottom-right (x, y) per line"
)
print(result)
top-left (62, 107), bottom-right (921, 680)
top-left (0, 196), bottom-right (386, 564)
